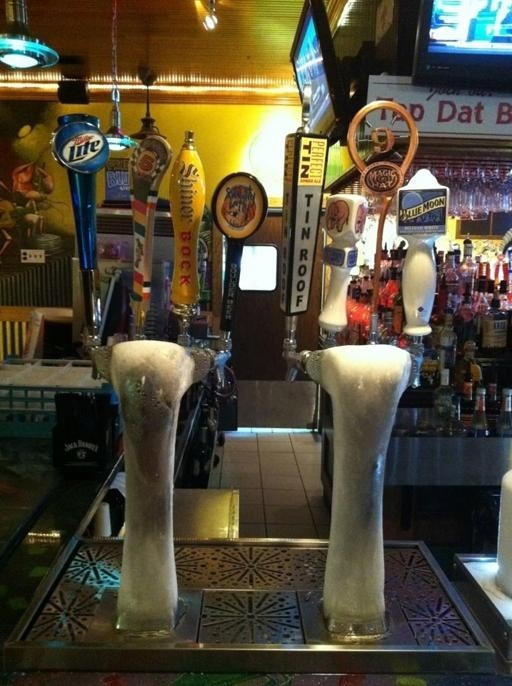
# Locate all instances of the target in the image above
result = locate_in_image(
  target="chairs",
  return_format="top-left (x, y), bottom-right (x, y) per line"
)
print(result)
top-left (51, 393), bottom-right (112, 492)
top-left (0, 305), bottom-right (45, 366)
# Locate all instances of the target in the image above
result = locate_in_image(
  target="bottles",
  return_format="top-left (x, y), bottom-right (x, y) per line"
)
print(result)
top-left (347, 243), bottom-right (512, 437)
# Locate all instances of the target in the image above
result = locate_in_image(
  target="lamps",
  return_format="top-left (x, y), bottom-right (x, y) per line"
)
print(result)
top-left (0, 0), bottom-right (60, 72)
top-left (91, 0), bottom-right (139, 152)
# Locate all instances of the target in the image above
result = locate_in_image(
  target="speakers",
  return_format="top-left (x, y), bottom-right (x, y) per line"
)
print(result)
top-left (57, 87), bottom-right (90, 104)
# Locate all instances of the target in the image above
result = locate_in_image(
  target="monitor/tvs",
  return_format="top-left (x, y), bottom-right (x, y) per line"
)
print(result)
top-left (289, 1), bottom-right (351, 146)
top-left (412, 1), bottom-right (511, 90)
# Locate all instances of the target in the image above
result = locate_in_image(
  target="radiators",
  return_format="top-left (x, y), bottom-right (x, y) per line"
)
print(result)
top-left (0, 252), bottom-right (73, 329)
